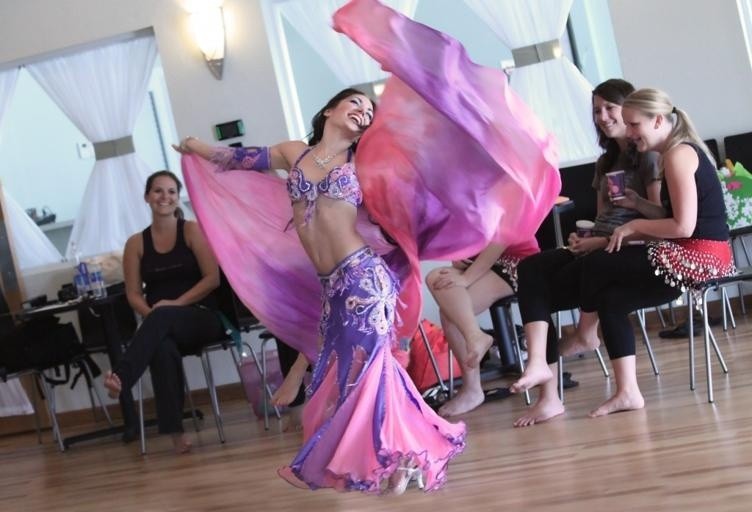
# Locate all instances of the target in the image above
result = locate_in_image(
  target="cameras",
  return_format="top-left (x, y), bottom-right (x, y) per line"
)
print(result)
top-left (58, 283), bottom-right (77, 301)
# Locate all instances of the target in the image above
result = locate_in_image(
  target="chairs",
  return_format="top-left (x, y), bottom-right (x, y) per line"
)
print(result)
top-left (535, 161), bottom-right (658, 378)
top-left (705, 138), bottom-right (746, 331)
top-left (448, 203), bottom-right (564, 409)
top-left (724, 132), bottom-right (748, 319)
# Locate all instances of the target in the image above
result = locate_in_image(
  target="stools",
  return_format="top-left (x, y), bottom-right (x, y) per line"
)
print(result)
top-left (138, 290), bottom-right (281, 458)
top-left (256, 329), bottom-right (291, 432)
top-left (0, 353), bottom-right (117, 452)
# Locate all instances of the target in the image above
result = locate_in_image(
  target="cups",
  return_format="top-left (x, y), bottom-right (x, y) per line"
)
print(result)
top-left (605, 171), bottom-right (625, 201)
top-left (57, 283), bottom-right (74, 303)
top-left (575, 220), bottom-right (594, 237)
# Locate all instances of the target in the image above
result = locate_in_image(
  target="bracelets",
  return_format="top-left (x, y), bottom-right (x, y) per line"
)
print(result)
top-left (181, 136), bottom-right (196, 153)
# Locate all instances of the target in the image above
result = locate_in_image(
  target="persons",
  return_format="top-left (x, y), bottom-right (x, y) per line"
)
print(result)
top-left (508, 79), bottom-right (663, 428)
top-left (104, 171), bottom-right (226, 453)
top-left (555, 89), bottom-right (733, 418)
top-left (426, 229), bottom-right (540, 418)
top-left (267, 339), bottom-right (312, 435)
top-left (171, 2), bottom-right (560, 499)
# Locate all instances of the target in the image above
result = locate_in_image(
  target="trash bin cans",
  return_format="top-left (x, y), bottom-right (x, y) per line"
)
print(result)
top-left (238, 349), bottom-right (291, 418)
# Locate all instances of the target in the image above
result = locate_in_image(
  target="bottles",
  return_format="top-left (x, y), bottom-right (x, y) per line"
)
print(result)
top-left (69, 241), bottom-right (107, 298)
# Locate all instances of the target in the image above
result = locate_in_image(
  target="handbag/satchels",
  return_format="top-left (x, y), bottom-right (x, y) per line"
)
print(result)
top-left (718, 158), bottom-right (751, 231)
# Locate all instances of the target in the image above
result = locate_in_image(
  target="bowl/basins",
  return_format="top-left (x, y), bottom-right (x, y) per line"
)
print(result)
top-left (30, 294), bottom-right (46, 308)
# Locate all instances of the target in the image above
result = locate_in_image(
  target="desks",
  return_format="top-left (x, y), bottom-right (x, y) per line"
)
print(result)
top-left (20, 282), bottom-right (203, 446)
top-left (687, 270), bottom-right (751, 406)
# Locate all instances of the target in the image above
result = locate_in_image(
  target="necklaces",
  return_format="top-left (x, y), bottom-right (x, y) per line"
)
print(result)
top-left (310, 149), bottom-right (347, 168)
